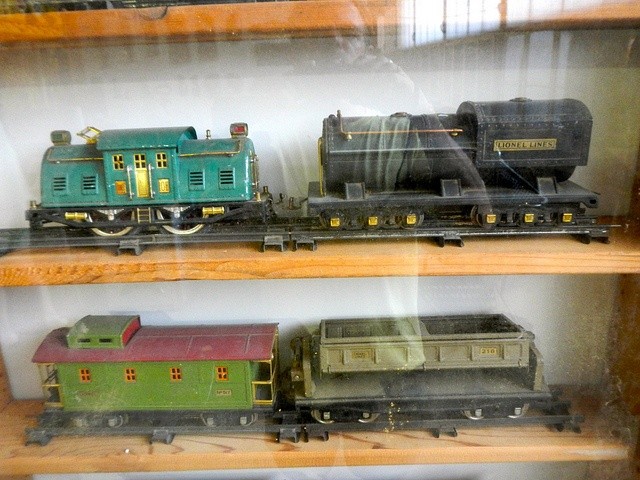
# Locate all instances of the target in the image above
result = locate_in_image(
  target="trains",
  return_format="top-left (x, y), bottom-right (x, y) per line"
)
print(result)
top-left (26, 97), bottom-right (600, 238)
top-left (32, 313), bottom-right (543, 428)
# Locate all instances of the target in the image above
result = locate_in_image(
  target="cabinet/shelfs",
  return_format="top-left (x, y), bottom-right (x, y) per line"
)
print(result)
top-left (1, 0), bottom-right (639, 473)
